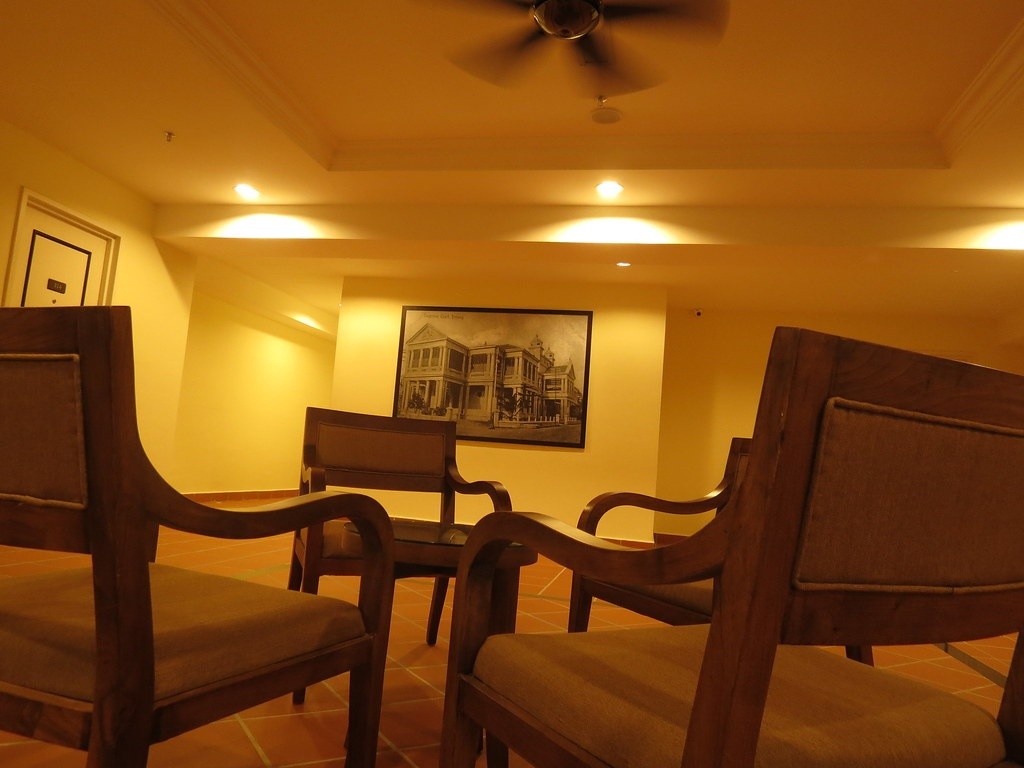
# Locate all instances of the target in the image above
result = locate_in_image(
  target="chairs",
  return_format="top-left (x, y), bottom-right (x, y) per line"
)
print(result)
top-left (0, 306), bottom-right (397, 768)
top-left (288, 407), bottom-right (539, 768)
top-left (567, 437), bottom-right (876, 668)
top-left (446, 327), bottom-right (1024, 768)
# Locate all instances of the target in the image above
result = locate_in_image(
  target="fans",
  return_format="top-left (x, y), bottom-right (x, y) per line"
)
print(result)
top-left (447, 0), bottom-right (731, 99)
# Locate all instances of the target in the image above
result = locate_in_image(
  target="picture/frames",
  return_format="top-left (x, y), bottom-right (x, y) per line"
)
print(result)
top-left (392, 306), bottom-right (594, 450)
top-left (21, 229), bottom-right (92, 306)
